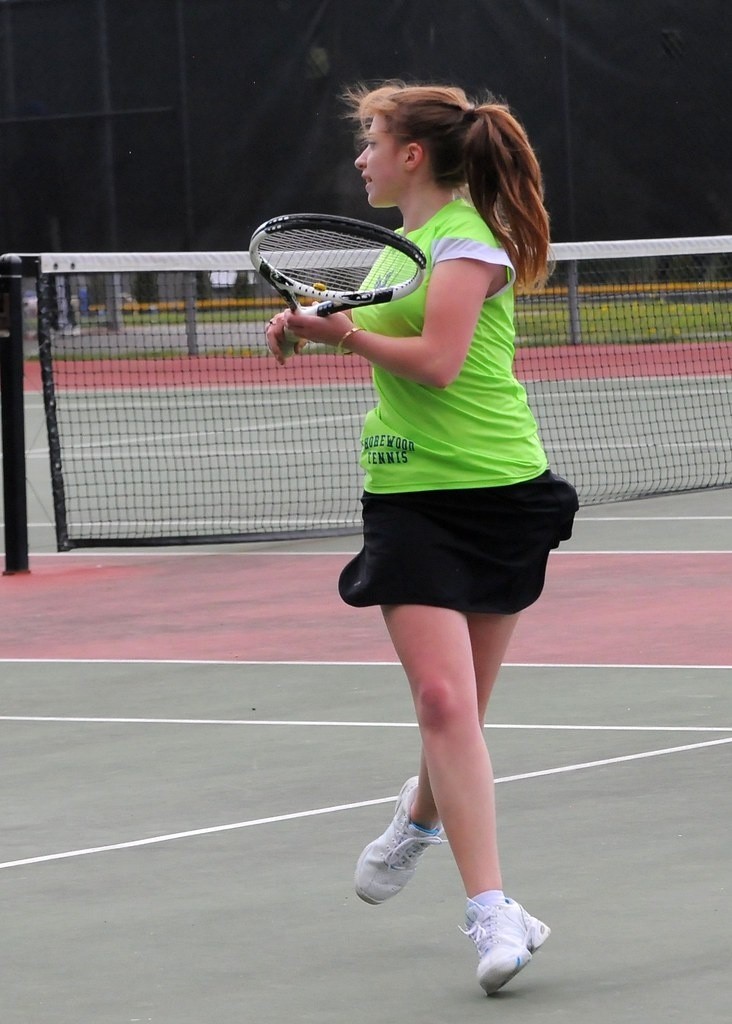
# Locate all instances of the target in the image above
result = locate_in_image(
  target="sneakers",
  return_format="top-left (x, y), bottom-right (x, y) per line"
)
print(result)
top-left (457, 894), bottom-right (551, 996)
top-left (355, 775), bottom-right (448, 906)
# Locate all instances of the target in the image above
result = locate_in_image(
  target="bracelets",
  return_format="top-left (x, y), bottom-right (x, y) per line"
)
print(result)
top-left (337, 327), bottom-right (368, 355)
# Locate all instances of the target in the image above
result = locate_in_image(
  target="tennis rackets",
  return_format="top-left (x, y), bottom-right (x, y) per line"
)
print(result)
top-left (249, 214), bottom-right (429, 360)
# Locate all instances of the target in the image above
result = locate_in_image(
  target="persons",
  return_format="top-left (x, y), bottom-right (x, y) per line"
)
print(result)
top-left (35, 215), bottom-right (83, 337)
top-left (334, 73), bottom-right (581, 996)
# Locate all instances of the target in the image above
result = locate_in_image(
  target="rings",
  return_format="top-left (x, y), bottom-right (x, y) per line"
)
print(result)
top-left (270, 319), bottom-right (276, 325)
